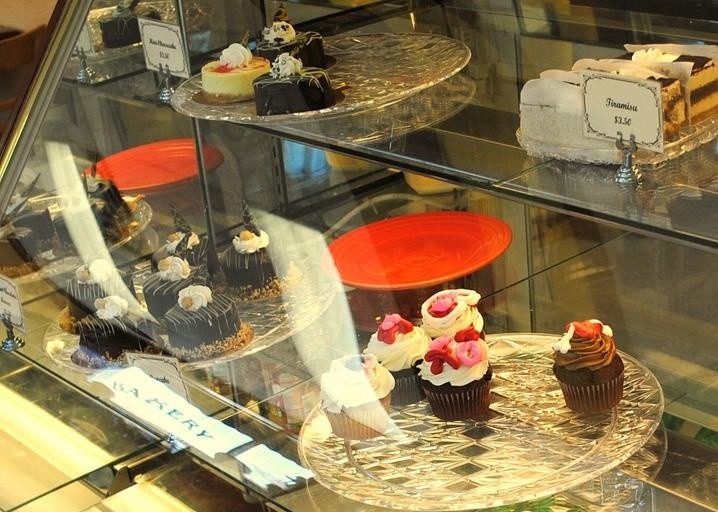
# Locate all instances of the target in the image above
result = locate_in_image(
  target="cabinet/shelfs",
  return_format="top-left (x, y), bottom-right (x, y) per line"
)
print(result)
top-left (0, 0), bottom-right (718, 512)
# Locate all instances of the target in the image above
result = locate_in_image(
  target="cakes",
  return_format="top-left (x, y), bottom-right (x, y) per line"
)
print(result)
top-left (319, 289), bottom-right (494, 440)
top-left (0, 149), bottom-right (136, 278)
top-left (549, 317), bottom-right (626, 415)
top-left (84, 0), bottom-right (161, 53)
top-left (199, 1), bottom-right (337, 117)
top-left (514, 43), bottom-right (718, 165)
top-left (63, 202), bottom-right (298, 371)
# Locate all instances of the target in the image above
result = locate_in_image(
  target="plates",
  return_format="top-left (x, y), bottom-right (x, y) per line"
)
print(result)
top-left (322, 74), bottom-right (478, 148)
top-left (561, 423), bottom-right (670, 509)
top-left (0, 189), bottom-right (154, 283)
top-left (167, 30), bottom-right (470, 124)
top-left (42, 243), bottom-right (334, 373)
top-left (294, 329), bottom-right (665, 509)
top-left (79, 137), bottom-right (223, 194)
top-left (324, 209), bottom-right (511, 292)
top-left (303, 429), bottom-right (670, 509)
